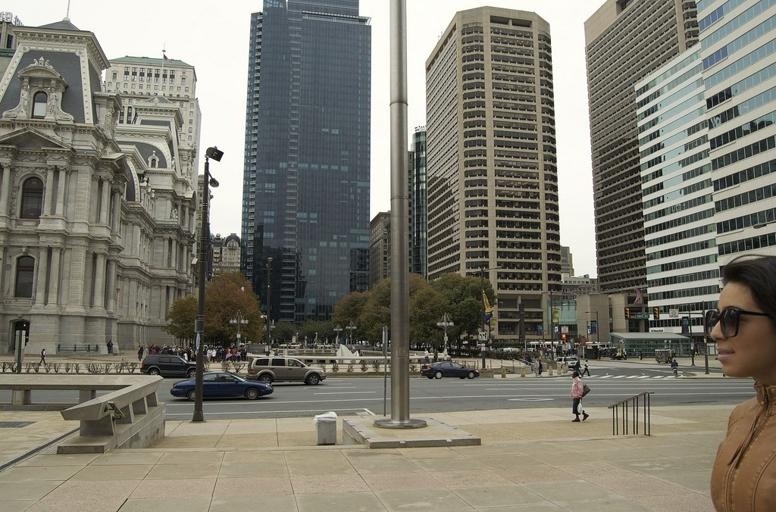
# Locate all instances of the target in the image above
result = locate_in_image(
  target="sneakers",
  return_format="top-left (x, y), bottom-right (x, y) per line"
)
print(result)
top-left (572, 419), bottom-right (580, 422)
top-left (583, 414), bottom-right (589, 420)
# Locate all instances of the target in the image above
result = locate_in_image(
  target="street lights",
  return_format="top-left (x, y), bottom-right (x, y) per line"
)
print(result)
top-left (189, 147), bottom-right (223, 419)
top-left (345, 320), bottom-right (357, 351)
top-left (313, 332), bottom-right (318, 342)
top-left (435, 312), bottom-right (454, 356)
top-left (263, 257), bottom-right (273, 352)
top-left (585, 311), bottom-right (600, 360)
top-left (540, 290), bottom-right (553, 344)
top-left (295, 332), bottom-right (298, 343)
top-left (332, 323), bottom-right (343, 344)
top-left (479, 265), bottom-right (502, 369)
top-left (228, 309), bottom-right (249, 350)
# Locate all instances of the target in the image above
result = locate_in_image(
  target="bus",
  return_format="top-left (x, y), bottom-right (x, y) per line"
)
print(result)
top-left (526, 342), bottom-right (570, 354)
top-left (245, 357), bottom-right (326, 385)
top-left (573, 341), bottom-right (611, 356)
top-left (491, 342), bottom-right (534, 358)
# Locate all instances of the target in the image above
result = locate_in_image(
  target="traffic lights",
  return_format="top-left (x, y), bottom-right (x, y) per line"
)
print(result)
top-left (652, 307), bottom-right (659, 319)
top-left (624, 307), bottom-right (630, 320)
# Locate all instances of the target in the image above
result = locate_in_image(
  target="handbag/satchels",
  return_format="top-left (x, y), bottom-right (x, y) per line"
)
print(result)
top-left (582, 385), bottom-right (590, 397)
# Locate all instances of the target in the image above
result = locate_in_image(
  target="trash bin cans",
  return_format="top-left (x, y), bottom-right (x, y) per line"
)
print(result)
top-left (314, 412), bottom-right (337, 445)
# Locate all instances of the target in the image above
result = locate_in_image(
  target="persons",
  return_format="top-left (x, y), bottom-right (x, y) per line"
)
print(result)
top-left (670, 358), bottom-right (679, 374)
top-left (570, 371), bottom-right (589, 423)
top-left (704, 252), bottom-right (776, 511)
top-left (38, 348), bottom-right (47, 366)
top-left (537, 345), bottom-right (592, 378)
top-left (135, 342), bottom-right (247, 363)
top-left (107, 339), bottom-right (114, 354)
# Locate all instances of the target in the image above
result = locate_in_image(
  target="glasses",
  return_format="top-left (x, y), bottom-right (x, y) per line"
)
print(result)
top-left (705, 306), bottom-right (768, 338)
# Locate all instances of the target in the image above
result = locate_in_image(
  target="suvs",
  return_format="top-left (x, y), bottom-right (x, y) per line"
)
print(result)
top-left (554, 357), bottom-right (577, 369)
top-left (140, 354), bottom-right (206, 379)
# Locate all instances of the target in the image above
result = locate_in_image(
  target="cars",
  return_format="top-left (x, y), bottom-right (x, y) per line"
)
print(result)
top-left (170, 371), bottom-right (273, 402)
top-left (421, 360), bottom-right (479, 380)
